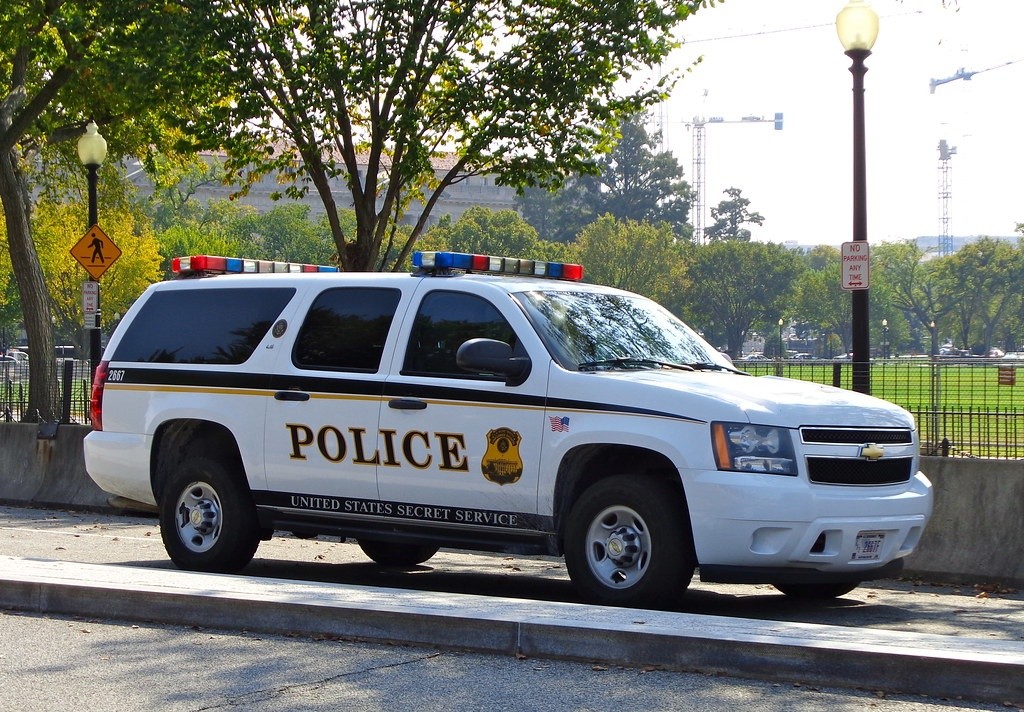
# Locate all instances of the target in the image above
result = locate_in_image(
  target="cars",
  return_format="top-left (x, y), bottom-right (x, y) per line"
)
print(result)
top-left (0, 355), bottom-right (22, 378)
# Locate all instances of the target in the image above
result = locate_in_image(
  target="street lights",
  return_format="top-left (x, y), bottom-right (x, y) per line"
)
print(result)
top-left (835, 4), bottom-right (880, 399)
top-left (77, 119), bottom-right (109, 396)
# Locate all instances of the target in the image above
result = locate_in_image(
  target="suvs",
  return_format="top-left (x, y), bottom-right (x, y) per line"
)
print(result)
top-left (82, 247), bottom-right (937, 612)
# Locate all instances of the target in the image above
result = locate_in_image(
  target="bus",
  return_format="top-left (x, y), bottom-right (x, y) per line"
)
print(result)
top-left (14, 346), bottom-right (75, 367)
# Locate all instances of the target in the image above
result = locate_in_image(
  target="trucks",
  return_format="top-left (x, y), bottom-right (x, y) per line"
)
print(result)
top-left (7, 351), bottom-right (28, 366)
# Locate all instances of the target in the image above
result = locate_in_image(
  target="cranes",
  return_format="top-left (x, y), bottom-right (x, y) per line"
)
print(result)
top-left (679, 110), bottom-right (784, 252)
top-left (929, 59), bottom-right (1020, 260)
